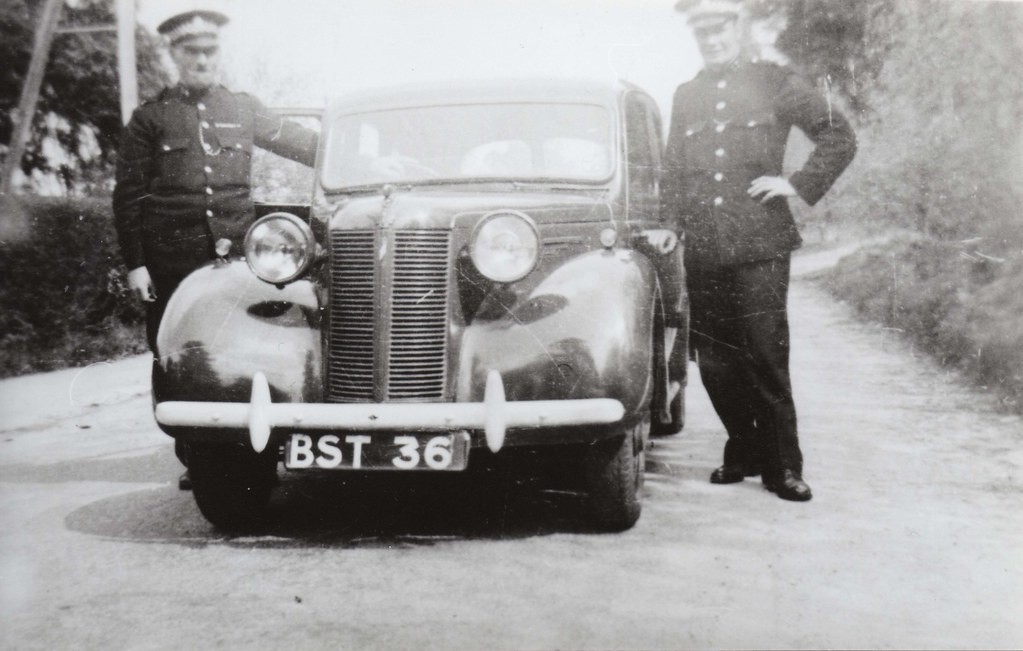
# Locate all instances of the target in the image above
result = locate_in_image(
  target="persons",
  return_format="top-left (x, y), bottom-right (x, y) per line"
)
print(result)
top-left (658, 0), bottom-right (856, 500)
top-left (112, 12), bottom-right (318, 489)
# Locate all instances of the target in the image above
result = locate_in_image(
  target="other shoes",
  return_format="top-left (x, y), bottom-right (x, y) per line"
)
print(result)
top-left (178, 468), bottom-right (192, 491)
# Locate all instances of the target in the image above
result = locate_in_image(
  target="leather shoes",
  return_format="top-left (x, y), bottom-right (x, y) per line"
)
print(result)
top-left (772, 469), bottom-right (812, 501)
top-left (711, 462), bottom-right (764, 483)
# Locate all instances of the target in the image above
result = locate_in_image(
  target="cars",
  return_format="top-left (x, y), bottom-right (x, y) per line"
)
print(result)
top-left (151, 79), bottom-right (690, 535)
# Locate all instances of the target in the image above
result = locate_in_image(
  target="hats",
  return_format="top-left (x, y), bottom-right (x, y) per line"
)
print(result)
top-left (157, 9), bottom-right (229, 49)
top-left (674, 0), bottom-right (745, 28)
